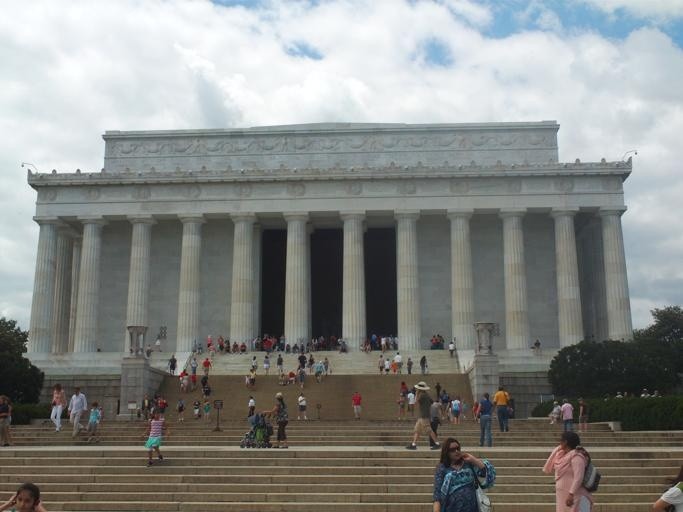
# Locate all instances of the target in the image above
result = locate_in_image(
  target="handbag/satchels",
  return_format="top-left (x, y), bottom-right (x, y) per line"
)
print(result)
top-left (474, 473), bottom-right (491, 511)
top-left (581, 452), bottom-right (601, 493)
top-left (508, 407), bottom-right (513, 418)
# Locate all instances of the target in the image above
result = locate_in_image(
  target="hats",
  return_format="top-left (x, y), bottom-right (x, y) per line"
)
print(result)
top-left (275, 392), bottom-right (283, 400)
top-left (414, 381), bottom-right (431, 391)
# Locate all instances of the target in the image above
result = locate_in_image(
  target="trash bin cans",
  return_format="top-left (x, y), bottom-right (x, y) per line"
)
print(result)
top-left (507, 399), bottom-right (515, 419)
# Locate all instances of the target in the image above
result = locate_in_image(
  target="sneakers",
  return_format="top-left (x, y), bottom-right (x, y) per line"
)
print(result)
top-left (159, 455), bottom-right (163, 462)
top-left (148, 461), bottom-right (156, 466)
top-left (406, 444), bottom-right (416, 450)
top-left (431, 443), bottom-right (441, 450)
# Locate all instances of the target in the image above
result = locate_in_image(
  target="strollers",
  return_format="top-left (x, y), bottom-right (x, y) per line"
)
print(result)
top-left (236, 409), bottom-right (273, 449)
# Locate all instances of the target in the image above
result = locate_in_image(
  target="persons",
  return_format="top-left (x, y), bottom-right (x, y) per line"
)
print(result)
top-left (0, 483), bottom-right (47, 512)
top-left (542, 432), bottom-right (593, 512)
top-left (432, 438), bottom-right (495, 512)
top-left (653, 464), bottom-right (683, 512)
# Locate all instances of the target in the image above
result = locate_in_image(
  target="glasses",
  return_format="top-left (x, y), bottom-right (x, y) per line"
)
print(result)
top-left (449, 446), bottom-right (460, 453)
